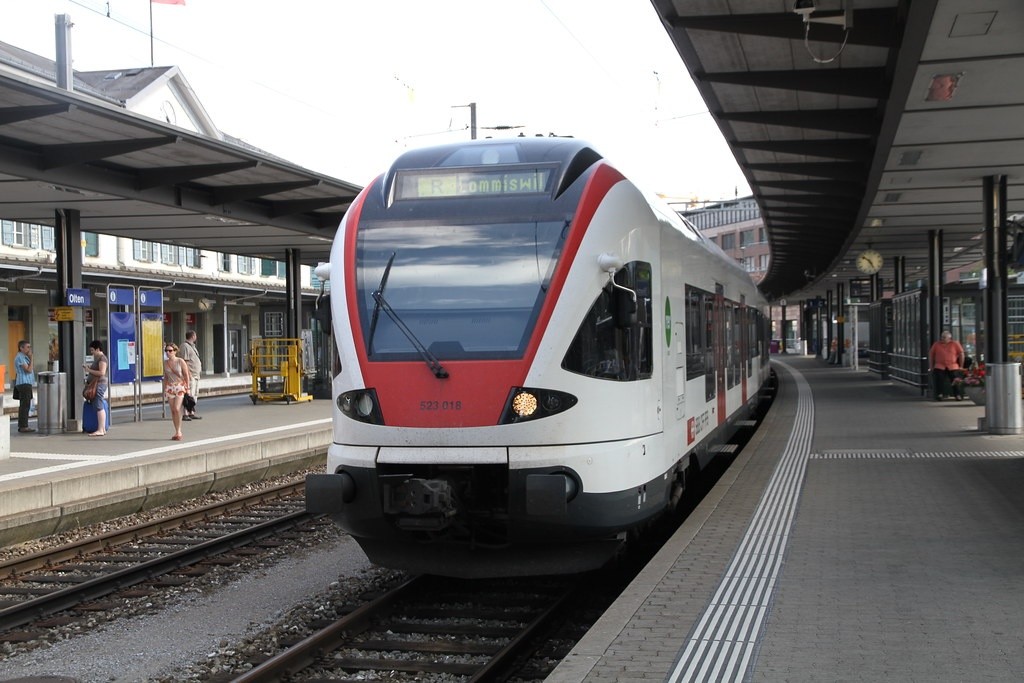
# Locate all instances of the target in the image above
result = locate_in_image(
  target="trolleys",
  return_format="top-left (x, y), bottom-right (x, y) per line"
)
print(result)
top-left (249, 336), bottom-right (313, 405)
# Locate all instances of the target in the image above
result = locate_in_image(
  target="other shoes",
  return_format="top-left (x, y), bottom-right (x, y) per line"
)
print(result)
top-left (88, 431), bottom-right (107, 436)
top-left (182, 415), bottom-right (191, 421)
top-left (936, 394), bottom-right (944, 401)
top-left (189, 414), bottom-right (202, 419)
top-left (172, 432), bottom-right (183, 441)
top-left (18, 427), bottom-right (36, 432)
top-left (956, 395), bottom-right (963, 400)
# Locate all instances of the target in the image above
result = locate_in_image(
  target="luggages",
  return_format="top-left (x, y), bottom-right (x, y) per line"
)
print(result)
top-left (82, 398), bottom-right (109, 433)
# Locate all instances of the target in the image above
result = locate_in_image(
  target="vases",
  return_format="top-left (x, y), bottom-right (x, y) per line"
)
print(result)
top-left (964, 386), bottom-right (987, 406)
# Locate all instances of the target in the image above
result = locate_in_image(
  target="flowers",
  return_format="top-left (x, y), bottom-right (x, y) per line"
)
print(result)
top-left (950, 363), bottom-right (992, 386)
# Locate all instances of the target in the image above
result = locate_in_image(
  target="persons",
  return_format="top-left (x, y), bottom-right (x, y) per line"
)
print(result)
top-left (828, 336), bottom-right (838, 364)
top-left (930, 331), bottom-right (965, 400)
top-left (162, 342), bottom-right (189, 441)
top-left (179, 330), bottom-right (202, 422)
top-left (14, 340), bottom-right (36, 432)
top-left (83, 340), bottom-right (109, 437)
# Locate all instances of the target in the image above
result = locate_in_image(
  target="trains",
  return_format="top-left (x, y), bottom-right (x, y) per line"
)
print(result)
top-left (306, 132), bottom-right (777, 579)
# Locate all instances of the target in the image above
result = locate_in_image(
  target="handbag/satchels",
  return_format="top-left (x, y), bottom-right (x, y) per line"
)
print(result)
top-left (13, 386), bottom-right (33, 400)
top-left (83, 377), bottom-right (98, 400)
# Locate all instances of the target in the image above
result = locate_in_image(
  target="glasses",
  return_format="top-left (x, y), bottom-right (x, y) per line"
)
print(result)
top-left (164, 349), bottom-right (174, 353)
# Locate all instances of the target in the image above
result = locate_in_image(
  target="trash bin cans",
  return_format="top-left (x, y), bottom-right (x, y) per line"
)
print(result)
top-left (770, 340), bottom-right (780, 353)
top-left (37, 371), bottom-right (68, 434)
top-left (984, 362), bottom-right (1024, 434)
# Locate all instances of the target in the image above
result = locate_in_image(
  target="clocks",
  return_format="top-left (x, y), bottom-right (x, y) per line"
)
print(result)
top-left (197, 298), bottom-right (210, 312)
top-left (856, 248), bottom-right (884, 276)
